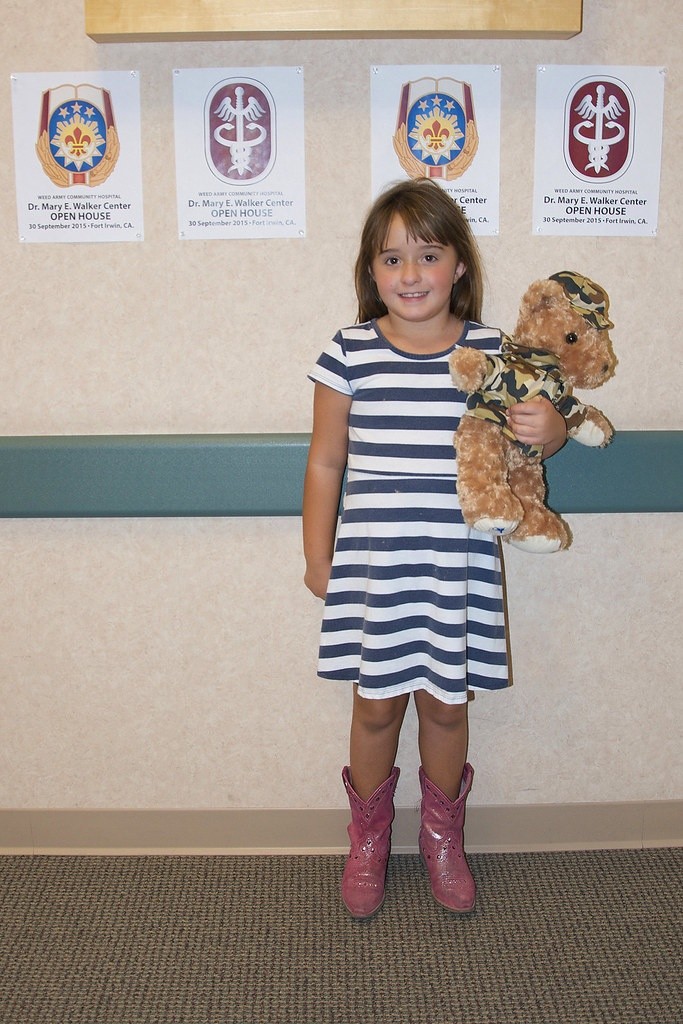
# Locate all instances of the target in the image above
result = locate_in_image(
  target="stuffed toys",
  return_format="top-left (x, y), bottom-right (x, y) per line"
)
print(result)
top-left (448, 270), bottom-right (619, 554)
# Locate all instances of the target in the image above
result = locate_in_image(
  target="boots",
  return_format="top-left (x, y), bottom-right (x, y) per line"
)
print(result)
top-left (341, 765), bottom-right (401, 919)
top-left (418, 764), bottom-right (476, 915)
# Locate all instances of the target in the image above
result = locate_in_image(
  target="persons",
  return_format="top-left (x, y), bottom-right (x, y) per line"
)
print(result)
top-left (301, 177), bottom-right (568, 920)
